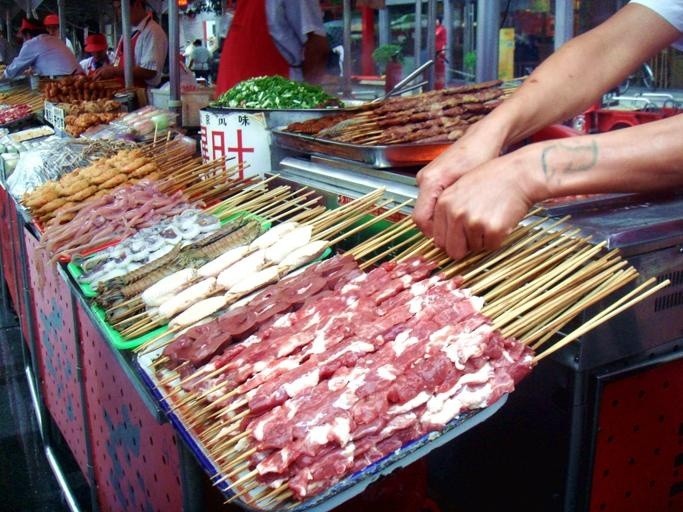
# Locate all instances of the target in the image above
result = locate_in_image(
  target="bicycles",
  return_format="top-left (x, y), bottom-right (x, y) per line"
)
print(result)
top-left (614, 60), bottom-right (660, 95)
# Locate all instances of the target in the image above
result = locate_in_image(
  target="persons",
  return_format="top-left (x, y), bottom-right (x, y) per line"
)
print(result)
top-left (0, 0), bottom-right (168, 89)
top-left (215, 0), bottom-right (327, 100)
top-left (433, 16), bottom-right (448, 90)
top-left (183, 34), bottom-right (226, 83)
top-left (412, 0), bottom-right (683, 261)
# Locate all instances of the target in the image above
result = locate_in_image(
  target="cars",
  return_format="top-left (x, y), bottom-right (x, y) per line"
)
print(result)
top-left (452, 23), bottom-right (539, 80)
top-left (390, 12), bottom-right (439, 48)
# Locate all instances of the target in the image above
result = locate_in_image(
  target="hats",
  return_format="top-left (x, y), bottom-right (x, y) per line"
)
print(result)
top-left (84, 33), bottom-right (107, 52)
top-left (43, 15), bottom-right (59, 25)
top-left (16, 19), bottom-right (37, 37)
